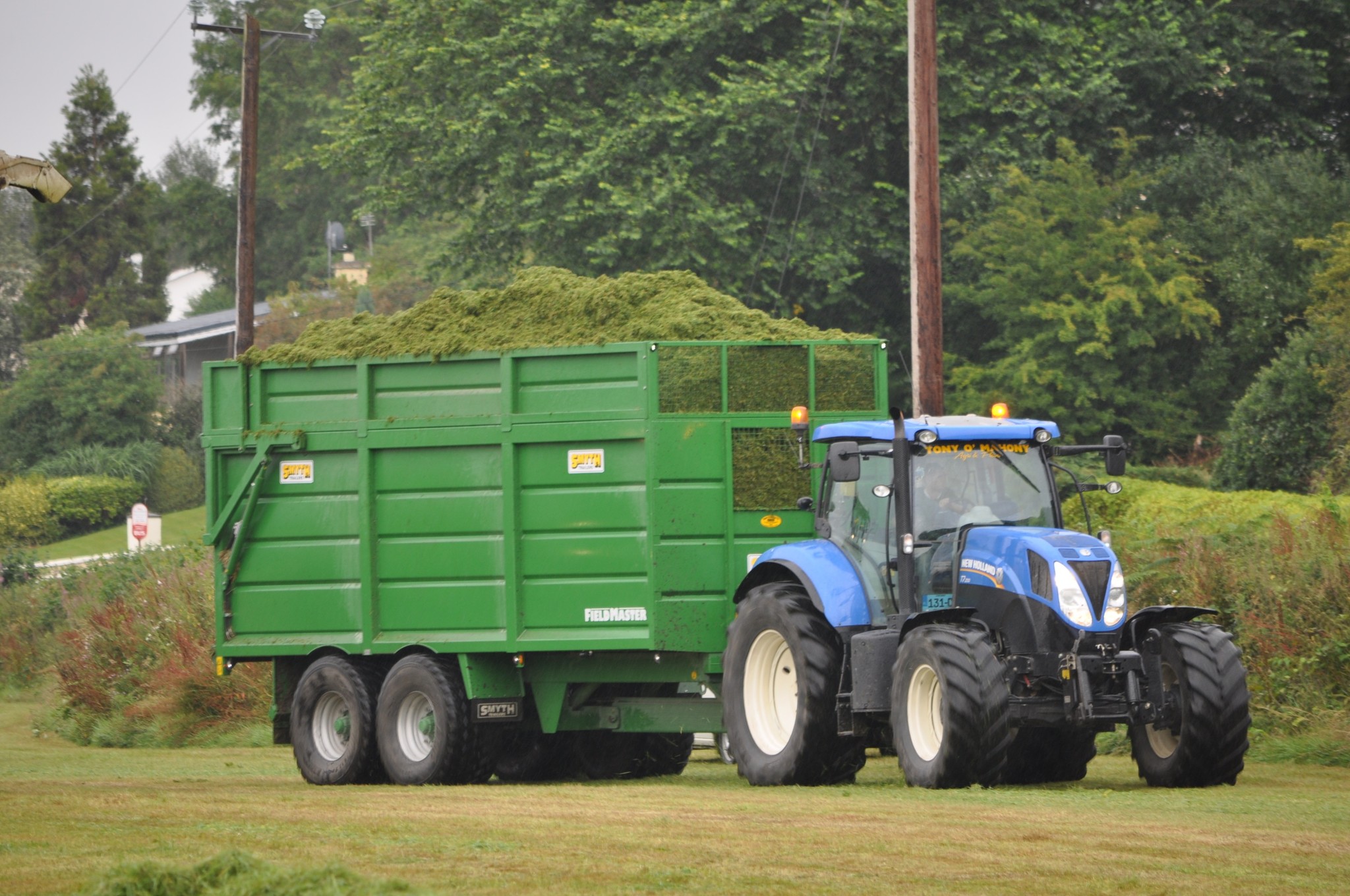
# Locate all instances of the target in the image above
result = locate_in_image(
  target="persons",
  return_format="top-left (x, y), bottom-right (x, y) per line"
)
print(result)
top-left (914, 462), bottom-right (974, 531)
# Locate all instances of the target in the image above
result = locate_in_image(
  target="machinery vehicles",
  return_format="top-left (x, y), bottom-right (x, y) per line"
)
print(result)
top-left (198, 339), bottom-right (1252, 790)
